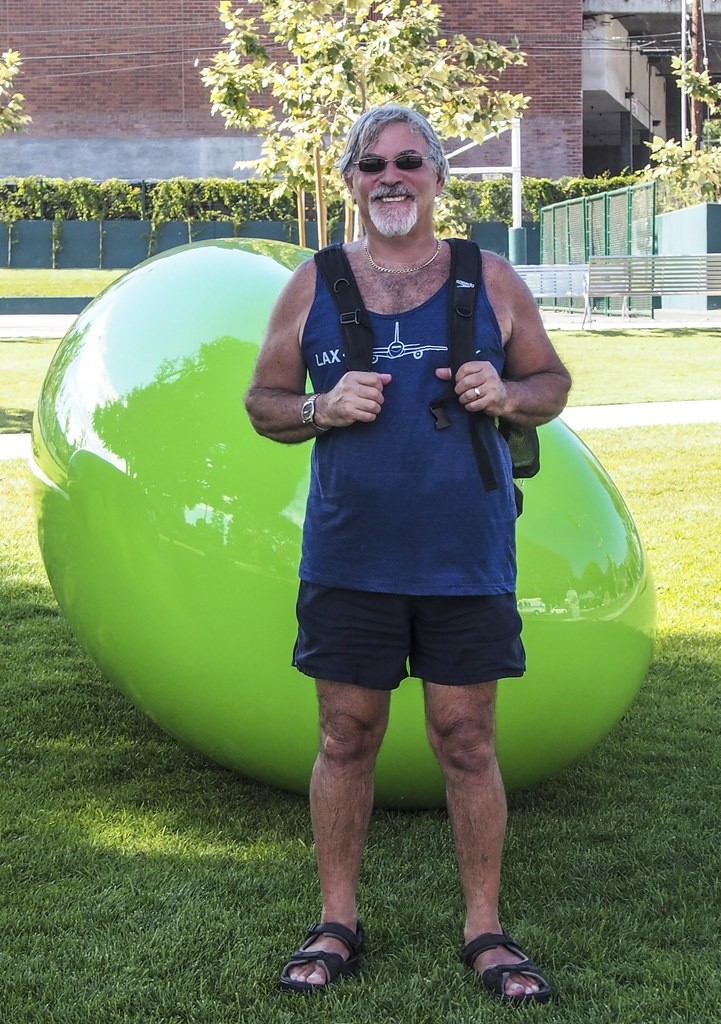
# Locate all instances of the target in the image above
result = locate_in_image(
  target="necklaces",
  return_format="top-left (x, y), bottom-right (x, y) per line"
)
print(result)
top-left (364, 238), bottom-right (442, 273)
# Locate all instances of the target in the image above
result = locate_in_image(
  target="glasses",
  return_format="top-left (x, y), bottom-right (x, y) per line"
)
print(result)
top-left (353, 154), bottom-right (431, 174)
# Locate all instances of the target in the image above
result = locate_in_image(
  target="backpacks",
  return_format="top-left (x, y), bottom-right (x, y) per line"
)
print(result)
top-left (313, 238), bottom-right (540, 478)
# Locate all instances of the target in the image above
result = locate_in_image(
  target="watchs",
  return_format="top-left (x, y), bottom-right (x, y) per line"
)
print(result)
top-left (301, 393), bottom-right (329, 434)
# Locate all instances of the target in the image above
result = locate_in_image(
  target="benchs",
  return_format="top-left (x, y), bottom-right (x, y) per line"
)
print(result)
top-left (512, 253), bottom-right (721, 331)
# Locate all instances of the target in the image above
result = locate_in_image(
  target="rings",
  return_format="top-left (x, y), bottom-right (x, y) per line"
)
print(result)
top-left (474, 388), bottom-right (481, 399)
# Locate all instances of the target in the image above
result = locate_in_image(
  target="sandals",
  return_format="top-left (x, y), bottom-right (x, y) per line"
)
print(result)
top-left (280, 920), bottom-right (365, 990)
top-left (462, 926), bottom-right (553, 1005)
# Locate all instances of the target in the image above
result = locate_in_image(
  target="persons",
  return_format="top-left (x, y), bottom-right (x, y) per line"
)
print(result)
top-left (244, 105), bottom-right (573, 1003)
